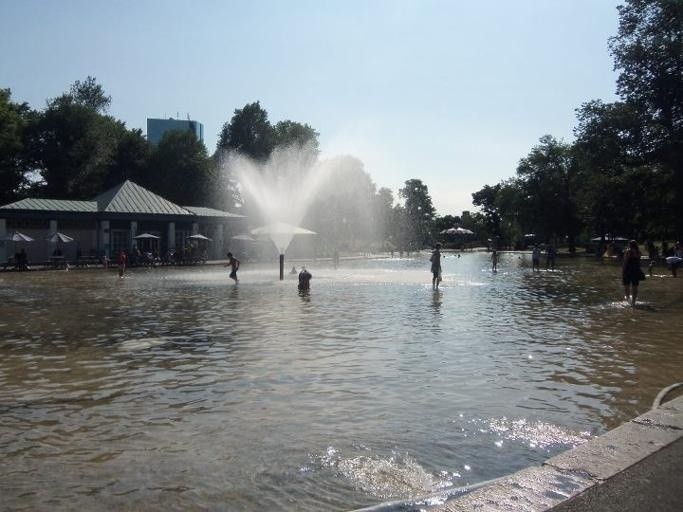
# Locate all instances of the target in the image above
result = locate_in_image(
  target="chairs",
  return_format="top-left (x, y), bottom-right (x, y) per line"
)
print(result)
top-left (2, 254), bottom-right (113, 271)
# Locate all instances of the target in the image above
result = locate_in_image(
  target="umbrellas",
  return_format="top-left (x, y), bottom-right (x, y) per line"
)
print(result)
top-left (1, 228), bottom-right (35, 253)
top-left (132, 231), bottom-right (160, 251)
top-left (185, 234), bottom-right (208, 247)
top-left (230, 234), bottom-right (256, 249)
top-left (45, 229), bottom-right (74, 248)
top-left (437, 225), bottom-right (473, 235)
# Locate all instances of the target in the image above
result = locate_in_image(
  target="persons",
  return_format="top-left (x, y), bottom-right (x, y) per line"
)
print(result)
top-left (299, 266), bottom-right (313, 297)
top-left (491, 250), bottom-right (499, 271)
top-left (675, 241), bottom-right (681, 256)
top-left (430, 243), bottom-right (443, 293)
top-left (546, 240), bottom-right (557, 269)
top-left (116, 249), bottom-right (126, 278)
top-left (623, 238), bottom-right (645, 306)
top-left (532, 243), bottom-right (541, 272)
top-left (289, 266), bottom-right (297, 273)
top-left (224, 252), bottom-right (241, 284)
top-left (663, 255), bottom-right (683, 278)
